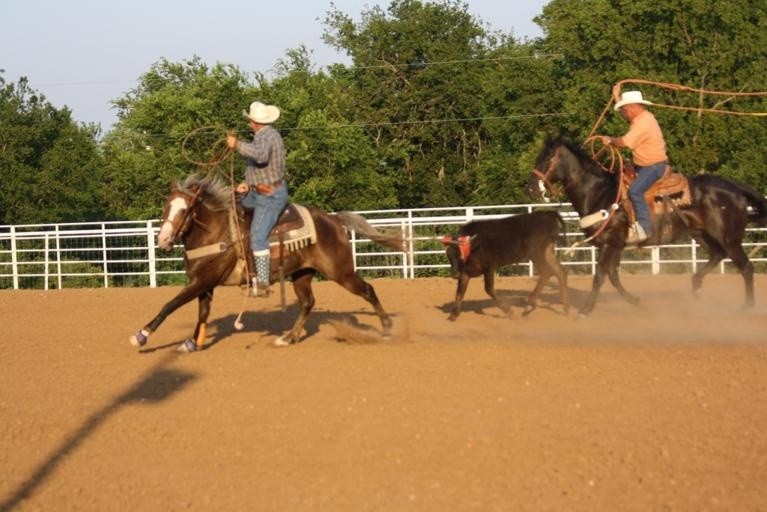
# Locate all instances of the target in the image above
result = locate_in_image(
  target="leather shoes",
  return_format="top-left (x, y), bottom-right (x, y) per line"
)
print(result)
top-left (638, 234), bottom-right (659, 248)
top-left (240, 285), bottom-right (270, 298)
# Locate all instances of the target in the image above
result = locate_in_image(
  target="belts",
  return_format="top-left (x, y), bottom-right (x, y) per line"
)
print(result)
top-left (248, 180), bottom-right (284, 193)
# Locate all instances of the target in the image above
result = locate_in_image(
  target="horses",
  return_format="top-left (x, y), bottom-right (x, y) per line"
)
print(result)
top-left (129, 173), bottom-right (410, 353)
top-left (525, 125), bottom-right (766, 319)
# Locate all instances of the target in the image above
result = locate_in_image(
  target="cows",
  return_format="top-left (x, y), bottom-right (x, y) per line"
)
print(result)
top-left (432, 209), bottom-right (569, 321)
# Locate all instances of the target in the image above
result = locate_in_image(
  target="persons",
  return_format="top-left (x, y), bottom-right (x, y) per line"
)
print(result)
top-left (601, 84), bottom-right (669, 244)
top-left (226, 101), bottom-right (288, 298)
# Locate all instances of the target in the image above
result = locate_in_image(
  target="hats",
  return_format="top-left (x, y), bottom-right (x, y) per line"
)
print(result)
top-left (241, 100), bottom-right (280, 125)
top-left (613, 91), bottom-right (652, 112)
